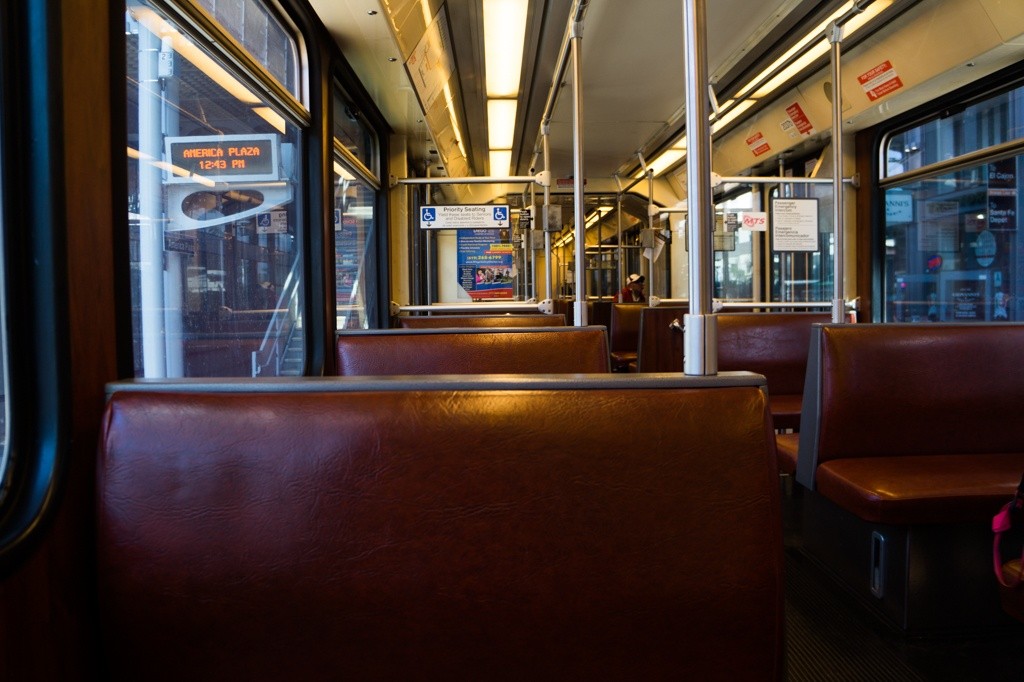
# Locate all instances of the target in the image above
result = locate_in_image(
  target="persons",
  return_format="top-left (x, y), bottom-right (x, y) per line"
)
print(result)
top-left (475, 268), bottom-right (512, 285)
top-left (613, 274), bottom-right (647, 305)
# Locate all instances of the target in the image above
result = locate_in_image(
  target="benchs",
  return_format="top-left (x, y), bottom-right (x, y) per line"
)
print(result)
top-left (629, 305), bottom-right (690, 374)
top-left (551, 294), bottom-right (611, 326)
top-left (800, 321), bottom-right (1024, 654)
top-left (397, 313), bottom-right (567, 329)
top-left (708, 306), bottom-right (852, 477)
top-left (606, 301), bottom-right (641, 372)
top-left (332, 325), bottom-right (612, 377)
top-left (92, 367), bottom-right (789, 682)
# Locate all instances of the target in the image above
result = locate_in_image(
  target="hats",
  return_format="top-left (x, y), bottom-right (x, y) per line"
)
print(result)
top-left (627, 274), bottom-right (645, 284)
